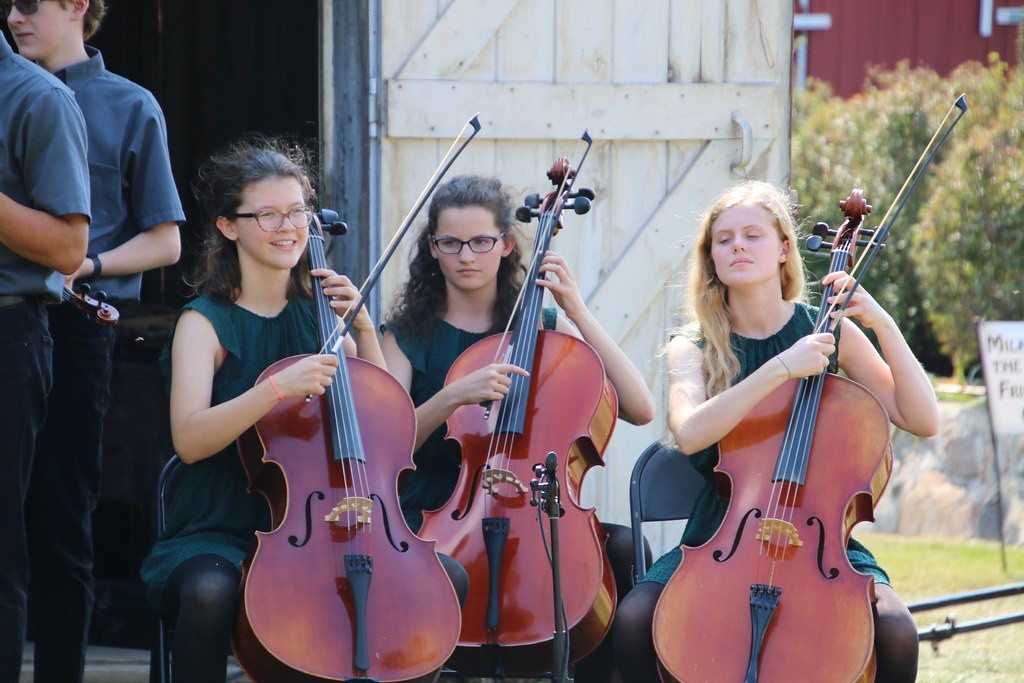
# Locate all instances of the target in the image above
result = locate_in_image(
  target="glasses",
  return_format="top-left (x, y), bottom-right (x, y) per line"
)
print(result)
top-left (0, 0), bottom-right (43, 19)
top-left (230, 203), bottom-right (314, 232)
top-left (430, 232), bottom-right (504, 253)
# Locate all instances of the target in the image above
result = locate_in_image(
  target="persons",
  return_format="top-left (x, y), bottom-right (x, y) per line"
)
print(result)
top-left (614, 178), bottom-right (941, 683)
top-left (133, 146), bottom-right (388, 683)
top-left (0, 0), bottom-right (186, 683)
top-left (379, 176), bottom-right (656, 683)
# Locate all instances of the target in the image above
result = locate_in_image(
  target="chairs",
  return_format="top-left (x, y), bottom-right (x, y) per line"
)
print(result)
top-left (148, 454), bottom-right (184, 683)
top-left (628, 431), bottom-right (708, 585)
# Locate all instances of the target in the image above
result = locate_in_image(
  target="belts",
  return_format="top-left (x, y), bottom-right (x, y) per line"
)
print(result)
top-left (0, 295), bottom-right (26, 308)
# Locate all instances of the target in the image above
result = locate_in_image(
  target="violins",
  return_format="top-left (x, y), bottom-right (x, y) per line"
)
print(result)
top-left (61, 281), bottom-right (121, 327)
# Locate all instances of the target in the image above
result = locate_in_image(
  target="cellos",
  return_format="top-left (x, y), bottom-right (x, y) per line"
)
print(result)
top-left (234, 143), bottom-right (466, 683)
top-left (648, 181), bottom-right (897, 683)
top-left (412, 154), bottom-right (620, 680)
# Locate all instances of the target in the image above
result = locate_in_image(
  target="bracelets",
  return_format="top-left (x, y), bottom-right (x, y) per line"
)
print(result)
top-left (776, 356), bottom-right (791, 381)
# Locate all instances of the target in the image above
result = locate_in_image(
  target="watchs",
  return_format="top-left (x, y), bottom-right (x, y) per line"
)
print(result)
top-left (85, 253), bottom-right (102, 276)
